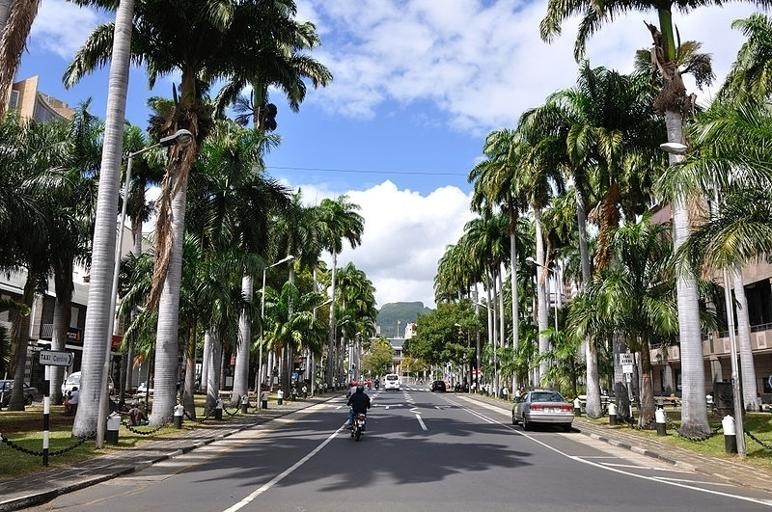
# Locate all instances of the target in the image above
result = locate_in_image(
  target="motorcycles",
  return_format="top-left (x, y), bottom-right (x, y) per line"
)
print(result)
top-left (350, 395), bottom-right (366, 443)
top-left (366, 378), bottom-right (379, 391)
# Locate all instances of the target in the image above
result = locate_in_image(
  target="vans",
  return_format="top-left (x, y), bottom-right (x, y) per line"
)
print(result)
top-left (384, 374), bottom-right (401, 391)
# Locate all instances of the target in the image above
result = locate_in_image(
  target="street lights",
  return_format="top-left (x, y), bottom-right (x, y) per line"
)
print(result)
top-left (95, 124), bottom-right (193, 450)
top-left (309, 299), bottom-right (360, 399)
top-left (658, 134), bottom-right (745, 455)
top-left (523, 254), bottom-right (566, 390)
top-left (454, 299), bottom-right (499, 395)
top-left (252, 254), bottom-right (295, 414)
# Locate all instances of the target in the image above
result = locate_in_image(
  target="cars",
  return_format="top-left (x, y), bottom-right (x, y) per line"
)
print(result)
top-left (510, 389), bottom-right (577, 432)
top-left (0, 379), bottom-right (39, 408)
top-left (431, 381), bottom-right (446, 393)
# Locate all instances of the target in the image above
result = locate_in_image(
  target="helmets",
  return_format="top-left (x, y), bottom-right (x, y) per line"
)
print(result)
top-left (350, 380), bottom-right (357, 386)
top-left (356, 385), bottom-right (364, 392)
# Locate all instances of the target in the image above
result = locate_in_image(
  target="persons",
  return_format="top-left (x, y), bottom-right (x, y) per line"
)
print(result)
top-left (346, 380), bottom-right (359, 425)
top-left (351, 378), bottom-right (373, 390)
top-left (455, 380), bottom-right (525, 400)
top-left (63, 387), bottom-right (79, 414)
top-left (67, 391), bottom-right (72, 399)
top-left (374, 379), bottom-right (380, 391)
top-left (347, 385), bottom-right (370, 438)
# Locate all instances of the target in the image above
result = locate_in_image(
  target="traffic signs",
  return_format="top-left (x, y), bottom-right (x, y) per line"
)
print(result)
top-left (37, 351), bottom-right (74, 366)
